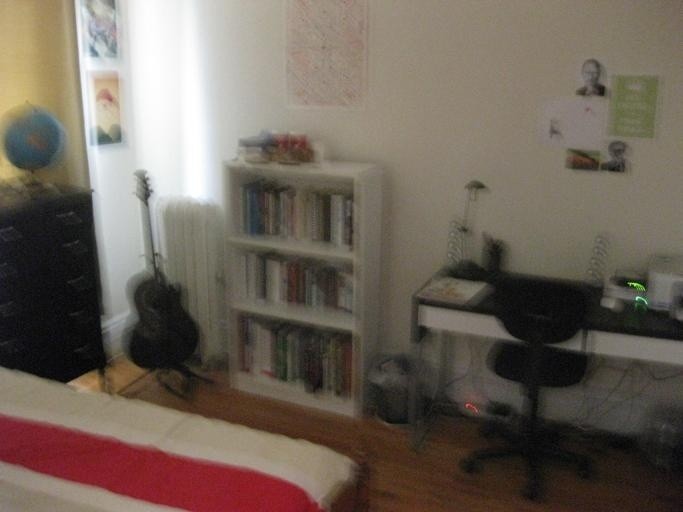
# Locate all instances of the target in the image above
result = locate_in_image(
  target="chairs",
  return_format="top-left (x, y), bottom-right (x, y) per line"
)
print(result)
top-left (457, 273), bottom-right (605, 499)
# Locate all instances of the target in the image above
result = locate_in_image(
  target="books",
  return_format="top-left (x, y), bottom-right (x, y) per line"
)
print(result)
top-left (416, 277), bottom-right (487, 308)
top-left (228, 171), bottom-right (354, 398)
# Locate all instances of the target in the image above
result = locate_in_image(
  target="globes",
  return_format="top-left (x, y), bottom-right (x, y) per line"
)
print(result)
top-left (0, 99), bottom-right (70, 194)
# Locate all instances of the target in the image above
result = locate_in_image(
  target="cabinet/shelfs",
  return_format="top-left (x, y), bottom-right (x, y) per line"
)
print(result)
top-left (0, 176), bottom-right (107, 392)
top-left (220, 158), bottom-right (383, 420)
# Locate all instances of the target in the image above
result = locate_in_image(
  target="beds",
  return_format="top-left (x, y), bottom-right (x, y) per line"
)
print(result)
top-left (0, 366), bottom-right (359, 512)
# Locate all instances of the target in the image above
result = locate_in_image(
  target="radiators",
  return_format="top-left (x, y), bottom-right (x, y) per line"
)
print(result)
top-left (155, 195), bottom-right (223, 371)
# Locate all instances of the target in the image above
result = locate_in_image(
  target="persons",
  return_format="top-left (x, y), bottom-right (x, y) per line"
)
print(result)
top-left (576, 58), bottom-right (607, 99)
top-left (600, 140), bottom-right (631, 175)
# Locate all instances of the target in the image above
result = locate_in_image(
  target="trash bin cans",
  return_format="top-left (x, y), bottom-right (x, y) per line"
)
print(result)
top-left (372, 353), bottom-right (424, 427)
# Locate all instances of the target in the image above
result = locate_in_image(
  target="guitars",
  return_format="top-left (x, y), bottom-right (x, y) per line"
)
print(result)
top-left (129, 172), bottom-right (197, 367)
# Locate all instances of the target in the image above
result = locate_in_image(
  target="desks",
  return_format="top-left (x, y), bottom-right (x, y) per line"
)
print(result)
top-left (407, 266), bottom-right (683, 454)
